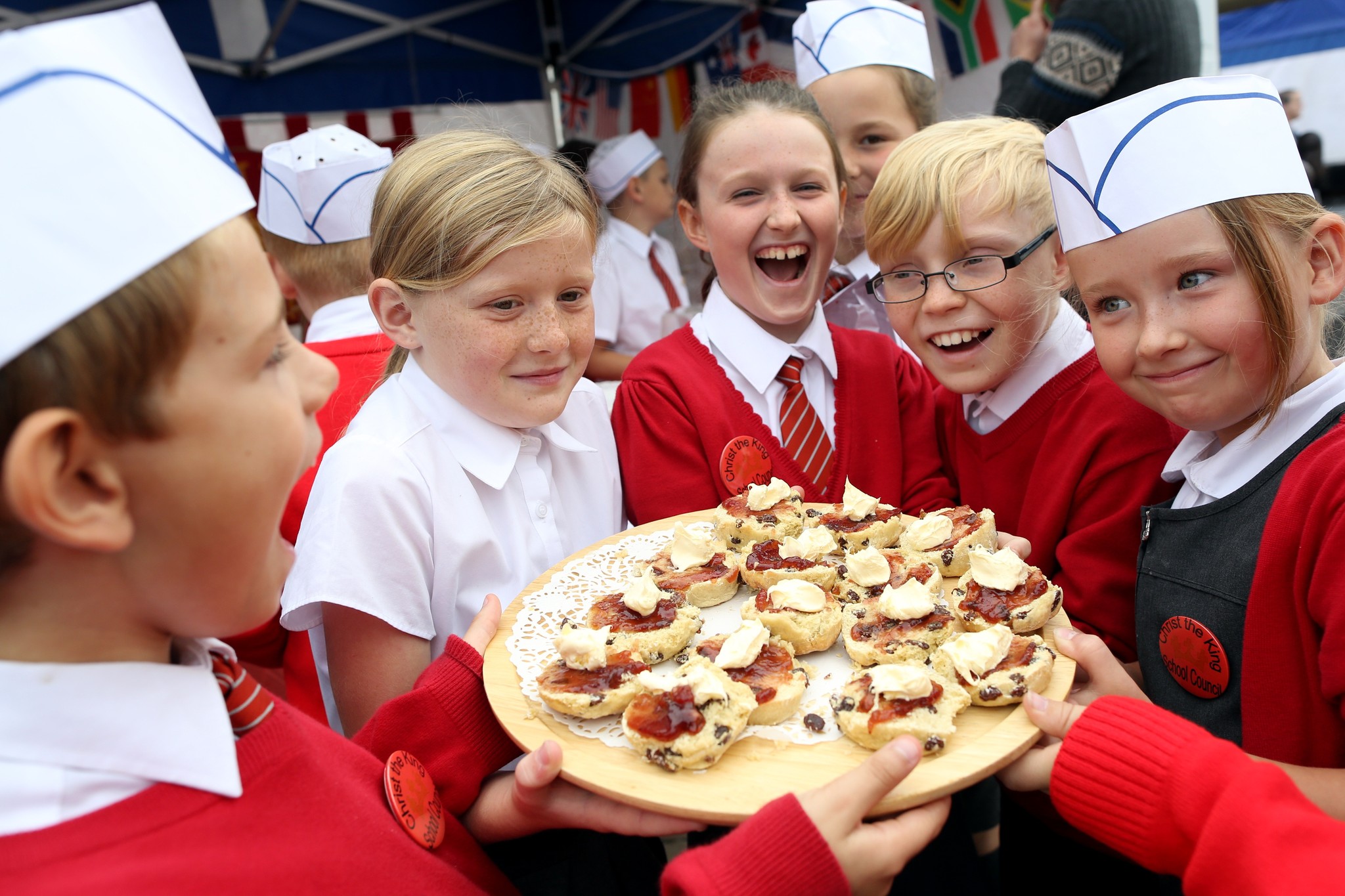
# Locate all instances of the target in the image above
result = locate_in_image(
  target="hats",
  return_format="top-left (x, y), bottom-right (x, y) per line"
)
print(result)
top-left (1042, 75), bottom-right (1317, 256)
top-left (586, 128), bottom-right (664, 207)
top-left (0, 0), bottom-right (258, 369)
top-left (257, 123), bottom-right (395, 246)
top-left (792, 1), bottom-right (936, 90)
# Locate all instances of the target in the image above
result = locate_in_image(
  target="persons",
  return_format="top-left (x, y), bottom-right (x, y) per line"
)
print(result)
top-left (0, 0), bottom-right (1345, 896)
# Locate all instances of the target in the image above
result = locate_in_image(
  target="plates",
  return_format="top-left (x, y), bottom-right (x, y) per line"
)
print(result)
top-left (479, 501), bottom-right (1081, 825)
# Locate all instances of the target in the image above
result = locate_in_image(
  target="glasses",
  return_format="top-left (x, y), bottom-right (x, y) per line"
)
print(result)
top-left (864, 223), bottom-right (1057, 305)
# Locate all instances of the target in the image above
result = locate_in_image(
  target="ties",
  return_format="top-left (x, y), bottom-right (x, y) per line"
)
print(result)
top-left (774, 356), bottom-right (836, 495)
top-left (209, 648), bottom-right (276, 737)
top-left (822, 275), bottom-right (852, 305)
top-left (648, 247), bottom-right (681, 310)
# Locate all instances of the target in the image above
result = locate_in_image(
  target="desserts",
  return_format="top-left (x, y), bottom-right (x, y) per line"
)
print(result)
top-left (539, 479), bottom-right (1064, 772)
